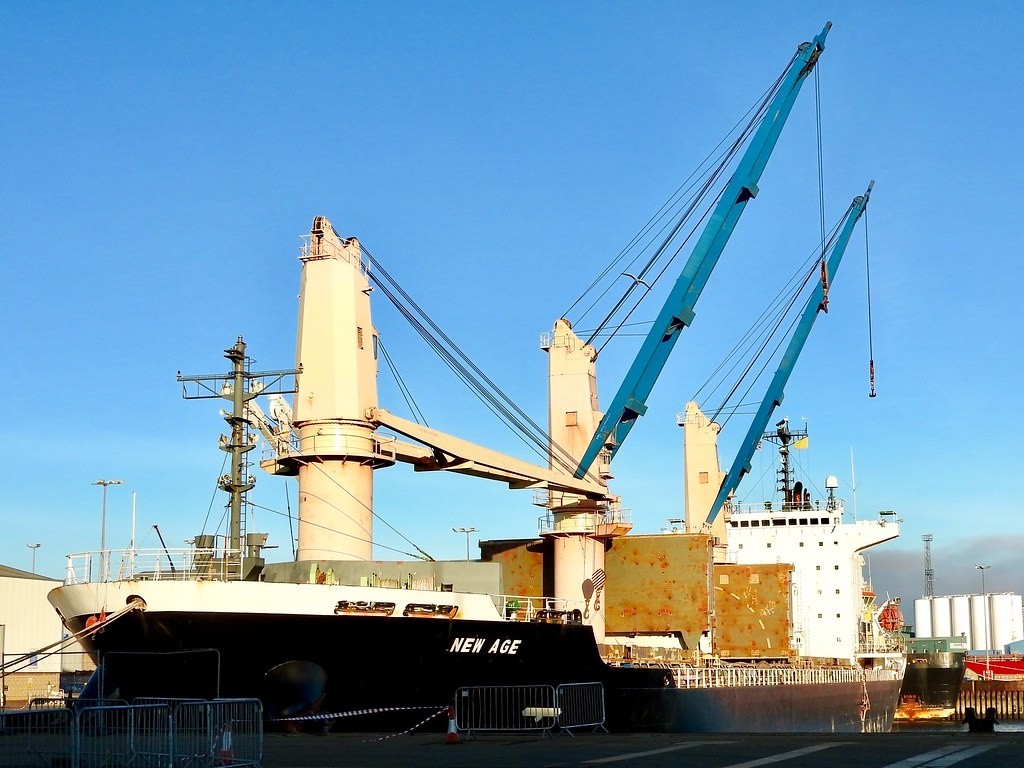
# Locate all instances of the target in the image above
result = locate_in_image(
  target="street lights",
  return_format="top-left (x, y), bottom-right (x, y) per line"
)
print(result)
top-left (89, 479), bottom-right (124, 583)
top-left (975, 563), bottom-right (991, 670)
top-left (26, 542), bottom-right (41, 574)
top-left (453, 527), bottom-right (480, 561)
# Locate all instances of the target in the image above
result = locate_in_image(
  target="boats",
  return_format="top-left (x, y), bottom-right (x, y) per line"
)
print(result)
top-left (46, 214), bottom-right (908, 735)
